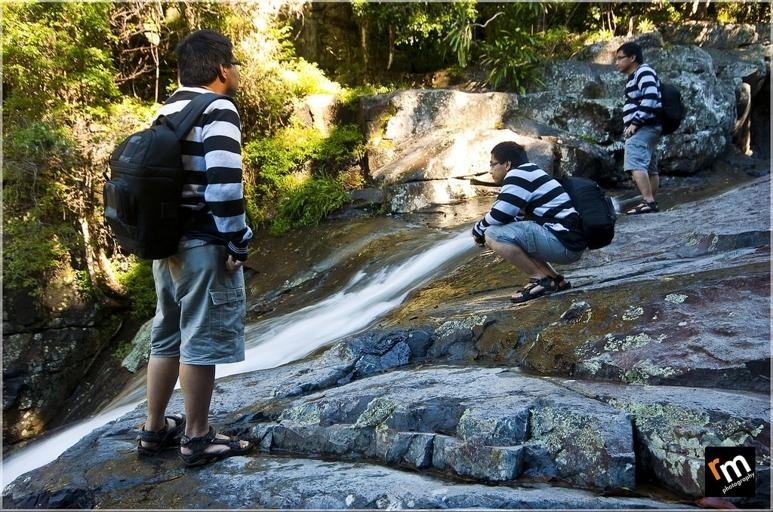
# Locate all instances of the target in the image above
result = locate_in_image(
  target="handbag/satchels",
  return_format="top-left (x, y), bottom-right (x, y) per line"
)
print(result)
top-left (553, 175), bottom-right (617, 252)
top-left (659, 83), bottom-right (685, 136)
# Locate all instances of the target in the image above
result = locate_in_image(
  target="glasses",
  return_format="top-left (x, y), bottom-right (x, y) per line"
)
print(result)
top-left (227, 59), bottom-right (240, 71)
top-left (614, 55), bottom-right (628, 62)
top-left (490, 159), bottom-right (504, 168)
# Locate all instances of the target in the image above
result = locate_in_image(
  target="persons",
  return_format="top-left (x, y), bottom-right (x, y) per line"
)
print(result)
top-left (614, 41), bottom-right (663, 215)
top-left (136, 28), bottom-right (257, 468)
top-left (471, 140), bottom-right (587, 304)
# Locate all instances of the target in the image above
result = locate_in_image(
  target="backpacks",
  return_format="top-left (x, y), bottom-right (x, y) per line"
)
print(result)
top-left (104, 93), bottom-right (239, 260)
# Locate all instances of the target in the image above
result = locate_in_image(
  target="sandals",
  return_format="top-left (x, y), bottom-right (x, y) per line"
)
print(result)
top-left (509, 272), bottom-right (572, 303)
top-left (138, 413), bottom-right (186, 454)
top-left (627, 198), bottom-right (660, 215)
top-left (178, 426), bottom-right (255, 467)
